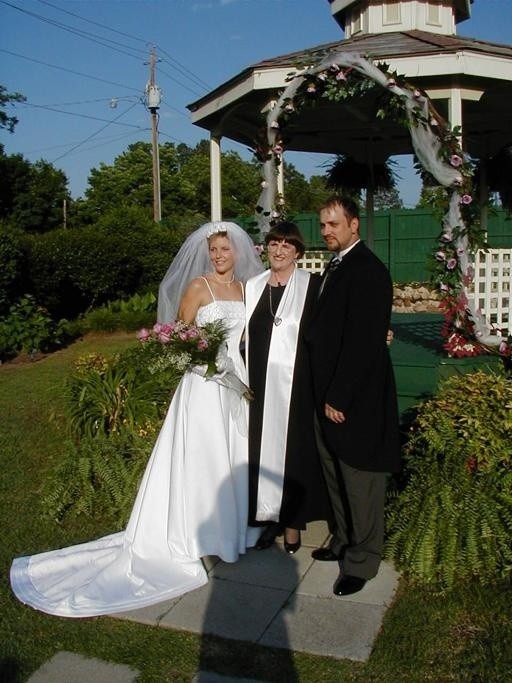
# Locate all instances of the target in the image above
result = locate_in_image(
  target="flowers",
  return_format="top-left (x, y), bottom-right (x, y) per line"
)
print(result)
top-left (133, 322), bottom-right (224, 372)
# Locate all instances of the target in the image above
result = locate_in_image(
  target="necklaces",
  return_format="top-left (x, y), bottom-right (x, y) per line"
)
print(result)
top-left (267, 261), bottom-right (298, 328)
top-left (210, 271), bottom-right (237, 285)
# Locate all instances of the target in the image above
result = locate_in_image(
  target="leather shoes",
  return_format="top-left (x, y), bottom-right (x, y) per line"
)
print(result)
top-left (311, 547), bottom-right (340, 561)
top-left (333, 572), bottom-right (366, 596)
top-left (284, 526), bottom-right (302, 554)
top-left (256, 523), bottom-right (283, 551)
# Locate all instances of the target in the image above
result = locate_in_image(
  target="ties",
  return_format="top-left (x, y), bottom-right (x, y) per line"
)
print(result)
top-left (324, 258), bottom-right (341, 291)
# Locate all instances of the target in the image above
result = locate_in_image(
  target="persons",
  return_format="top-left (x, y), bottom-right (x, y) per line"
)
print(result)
top-left (243, 221), bottom-right (397, 555)
top-left (170, 225), bottom-right (255, 565)
top-left (306, 193), bottom-right (403, 601)
